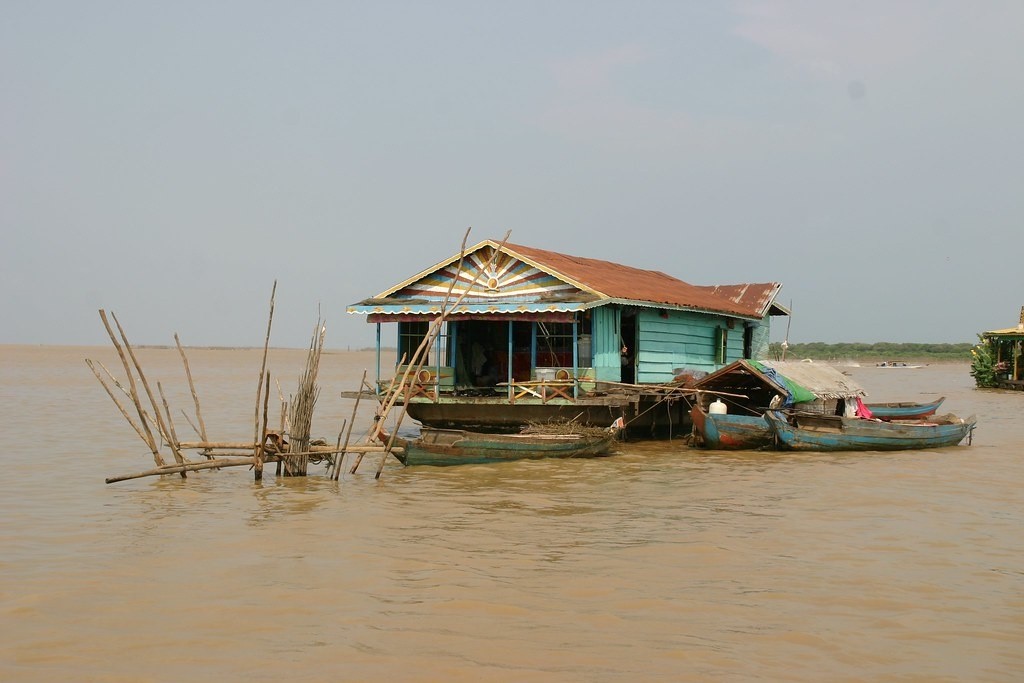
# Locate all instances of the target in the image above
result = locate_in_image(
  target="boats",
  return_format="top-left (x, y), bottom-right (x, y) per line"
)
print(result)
top-left (764, 406), bottom-right (976, 452)
top-left (865, 396), bottom-right (946, 419)
top-left (687, 357), bottom-right (867, 447)
top-left (374, 417), bottom-right (628, 466)
